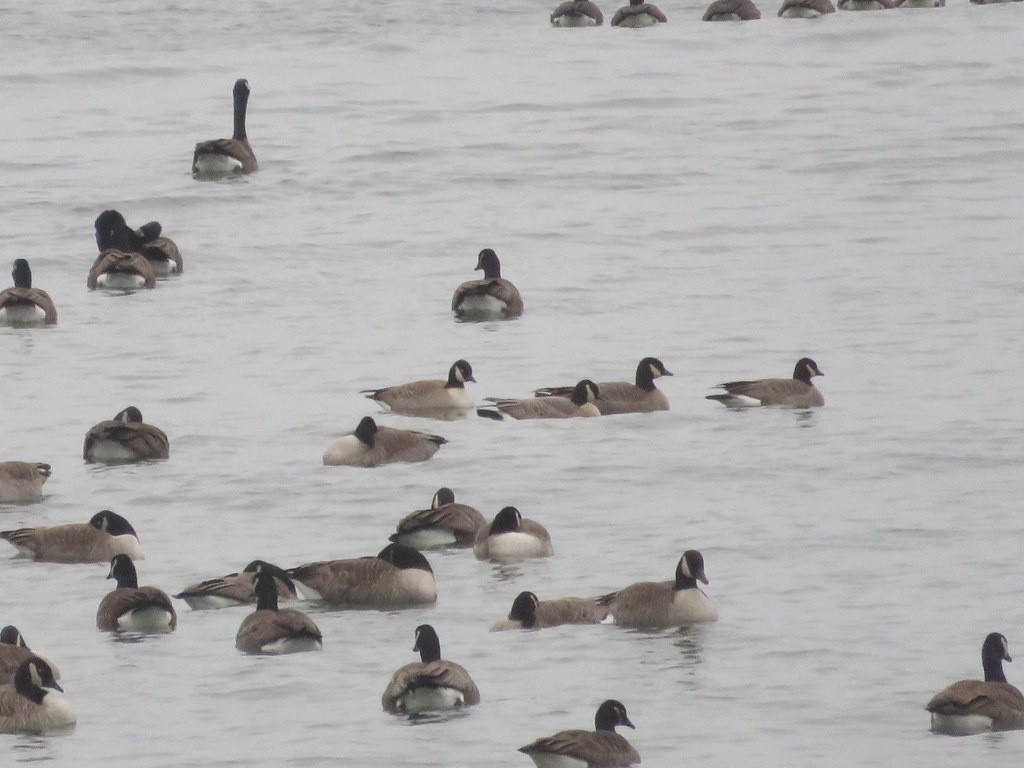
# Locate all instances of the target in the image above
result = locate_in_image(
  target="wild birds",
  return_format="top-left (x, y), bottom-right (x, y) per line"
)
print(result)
top-left (86, 209), bottom-right (184, 290)
top-left (451, 248), bottom-right (524, 316)
top-left (924, 632), bottom-right (1024, 733)
top-left (0, 258), bottom-right (57, 324)
top-left (1, 356), bottom-right (826, 768)
top-left (549, 0), bottom-right (946, 28)
top-left (191, 78), bottom-right (259, 175)
